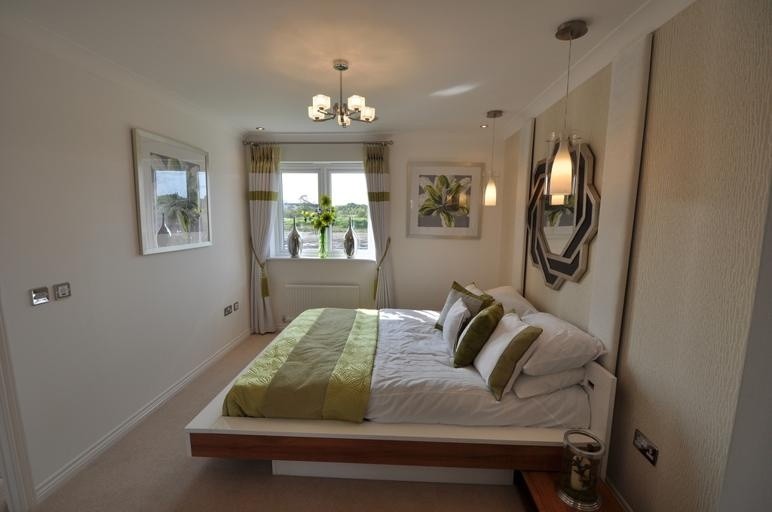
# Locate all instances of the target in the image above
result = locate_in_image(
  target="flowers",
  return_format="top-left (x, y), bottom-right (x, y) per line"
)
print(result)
top-left (301, 195), bottom-right (336, 253)
top-left (169, 193), bottom-right (202, 233)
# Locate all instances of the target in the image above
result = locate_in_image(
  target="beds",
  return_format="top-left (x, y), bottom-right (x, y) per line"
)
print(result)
top-left (185, 308), bottom-right (618, 486)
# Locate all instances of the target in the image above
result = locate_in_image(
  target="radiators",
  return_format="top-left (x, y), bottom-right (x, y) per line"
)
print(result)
top-left (283, 285), bottom-right (360, 322)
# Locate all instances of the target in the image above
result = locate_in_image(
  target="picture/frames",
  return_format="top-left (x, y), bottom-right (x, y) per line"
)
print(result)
top-left (406, 161), bottom-right (484, 240)
top-left (543, 196), bottom-right (575, 238)
top-left (131, 127), bottom-right (213, 255)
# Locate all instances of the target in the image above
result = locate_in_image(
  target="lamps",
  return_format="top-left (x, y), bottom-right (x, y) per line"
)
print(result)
top-left (551, 195), bottom-right (564, 206)
top-left (308, 60), bottom-right (375, 126)
top-left (484, 109), bottom-right (503, 206)
top-left (544, 20), bottom-right (589, 196)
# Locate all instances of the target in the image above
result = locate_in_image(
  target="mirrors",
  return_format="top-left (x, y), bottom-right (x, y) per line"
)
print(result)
top-left (524, 143), bottom-right (601, 289)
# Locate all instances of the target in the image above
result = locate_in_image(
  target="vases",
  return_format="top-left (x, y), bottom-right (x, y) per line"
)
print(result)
top-left (319, 227), bottom-right (326, 259)
top-left (287, 217), bottom-right (302, 258)
top-left (157, 213), bottom-right (170, 247)
top-left (343, 217), bottom-right (357, 258)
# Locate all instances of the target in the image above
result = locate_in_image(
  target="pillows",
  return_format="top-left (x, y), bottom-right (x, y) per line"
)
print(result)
top-left (473, 308), bottom-right (542, 401)
top-left (486, 286), bottom-right (537, 320)
top-left (444, 297), bottom-right (472, 357)
top-left (520, 312), bottom-right (607, 376)
top-left (514, 369), bottom-right (585, 399)
top-left (454, 302), bottom-right (503, 368)
top-left (435, 282), bottom-right (482, 332)
top-left (465, 281), bottom-right (494, 309)
